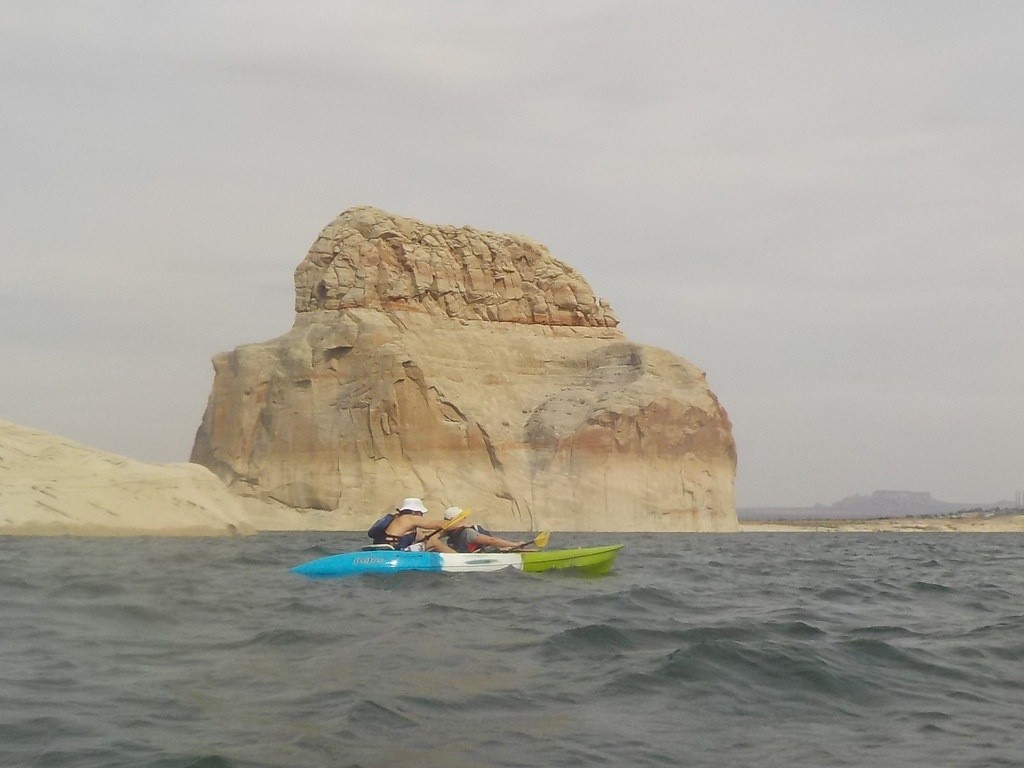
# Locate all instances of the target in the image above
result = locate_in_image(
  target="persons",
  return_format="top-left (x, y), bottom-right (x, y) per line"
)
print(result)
top-left (373, 497), bottom-right (464, 554)
top-left (444, 507), bottom-right (538, 553)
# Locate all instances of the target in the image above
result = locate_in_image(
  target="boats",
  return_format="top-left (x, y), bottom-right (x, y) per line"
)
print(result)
top-left (289, 538), bottom-right (624, 575)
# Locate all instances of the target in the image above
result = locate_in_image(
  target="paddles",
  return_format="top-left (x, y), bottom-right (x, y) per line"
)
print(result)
top-left (505, 528), bottom-right (552, 553)
top-left (415, 508), bottom-right (471, 544)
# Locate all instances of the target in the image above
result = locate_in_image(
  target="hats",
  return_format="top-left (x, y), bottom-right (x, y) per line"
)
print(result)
top-left (444, 507), bottom-right (464, 520)
top-left (399, 498), bottom-right (428, 513)
